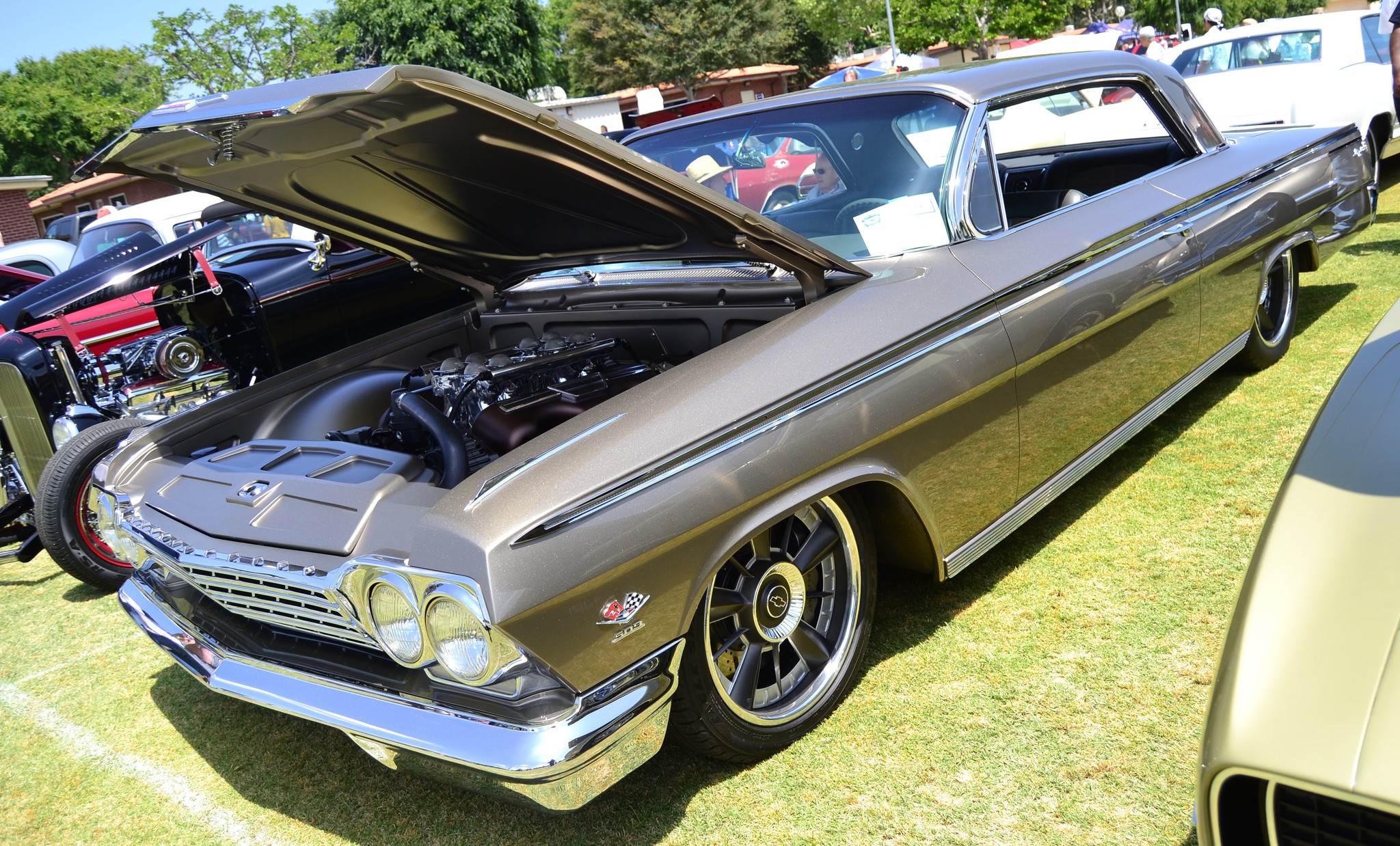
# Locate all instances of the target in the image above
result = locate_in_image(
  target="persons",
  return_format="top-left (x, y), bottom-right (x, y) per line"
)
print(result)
top-left (1389, 1), bottom-right (1400, 124)
top-left (896, 66), bottom-right (909, 73)
top-left (1198, 8), bottom-right (1238, 74)
top-left (1119, 27), bottom-right (1195, 55)
top-left (1140, 26), bottom-right (1166, 61)
top-left (1240, 7), bottom-right (1324, 67)
top-left (601, 125), bottom-right (608, 133)
top-left (901, 50), bottom-right (917, 57)
top-left (686, 155), bottom-right (733, 196)
top-left (807, 153), bottom-right (847, 199)
top-left (844, 70), bottom-right (857, 82)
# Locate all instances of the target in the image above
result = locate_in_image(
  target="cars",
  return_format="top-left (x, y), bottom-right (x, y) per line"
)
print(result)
top-left (0, 93), bottom-right (829, 593)
top-left (68, 54), bottom-right (1388, 814)
top-left (1186, 302), bottom-right (1400, 846)
top-left (900, 10), bottom-right (1400, 168)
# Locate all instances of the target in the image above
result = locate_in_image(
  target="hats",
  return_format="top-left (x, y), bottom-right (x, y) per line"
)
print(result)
top-left (686, 155), bottom-right (733, 184)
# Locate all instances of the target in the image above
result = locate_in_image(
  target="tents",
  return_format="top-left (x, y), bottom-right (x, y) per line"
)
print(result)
top-left (865, 48), bottom-right (939, 72)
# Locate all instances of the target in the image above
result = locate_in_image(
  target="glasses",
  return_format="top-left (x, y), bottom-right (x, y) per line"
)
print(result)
top-left (812, 168), bottom-right (831, 174)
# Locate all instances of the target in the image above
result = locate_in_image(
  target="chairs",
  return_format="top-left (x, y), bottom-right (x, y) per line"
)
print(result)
top-left (1030, 139), bottom-right (1186, 223)
top-left (867, 158), bottom-right (1012, 237)
top-left (1291, 42), bottom-right (1314, 62)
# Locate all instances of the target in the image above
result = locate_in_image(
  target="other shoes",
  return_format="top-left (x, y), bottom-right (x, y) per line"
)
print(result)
top-left (1316, 214), bottom-right (1374, 244)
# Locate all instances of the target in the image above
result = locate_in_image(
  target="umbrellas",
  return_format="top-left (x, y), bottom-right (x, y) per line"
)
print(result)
top-left (808, 66), bottom-right (888, 88)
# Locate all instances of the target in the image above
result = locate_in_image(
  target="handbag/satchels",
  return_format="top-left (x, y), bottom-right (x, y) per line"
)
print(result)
top-left (1267, 50), bottom-right (1280, 61)
